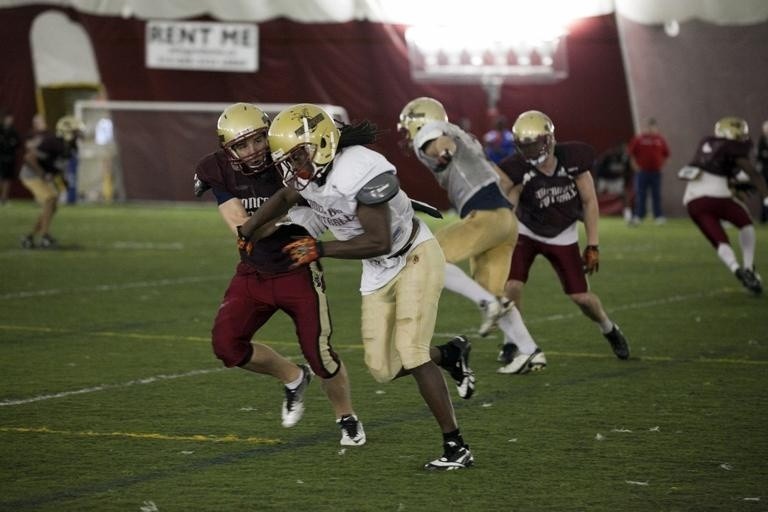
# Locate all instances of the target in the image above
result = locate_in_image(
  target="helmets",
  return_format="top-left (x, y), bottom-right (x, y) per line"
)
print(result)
top-left (55, 116), bottom-right (87, 144)
top-left (511, 110), bottom-right (555, 167)
top-left (216, 102), bottom-right (273, 176)
top-left (715, 117), bottom-right (751, 143)
top-left (397, 96), bottom-right (449, 159)
top-left (267, 102), bottom-right (342, 191)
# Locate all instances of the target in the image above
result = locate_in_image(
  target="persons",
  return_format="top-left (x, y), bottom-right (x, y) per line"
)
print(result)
top-left (18, 116), bottom-right (85, 248)
top-left (190, 101), bottom-right (366, 446)
top-left (499, 110), bottom-right (631, 362)
top-left (625, 117), bottom-right (671, 228)
top-left (595, 140), bottom-right (630, 221)
top-left (236, 103), bottom-right (476, 472)
top-left (395, 95), bottom-right (547, 375)
top-left (481, 116), bottom-right (512, 171)
top-left (678, 117), bottom-right (762, 294)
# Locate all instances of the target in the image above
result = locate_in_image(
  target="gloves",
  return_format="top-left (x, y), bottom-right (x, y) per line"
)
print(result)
top-left (287, 205), bottom-right (326, 241)
top-left (581, 246), bottom-right (599, 275)
top-left (237, 225), bottom-right (255, 261)
top-left (280, 235), bottom-right (325, 275)
top-left (433, 148), bottom-right (451, 171)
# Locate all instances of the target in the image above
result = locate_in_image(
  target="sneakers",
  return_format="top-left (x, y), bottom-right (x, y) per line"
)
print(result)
top-left (424, 438), bottom-right (475, 472)
top-left (449, 335), bottom-right (476, 399)
top-left (478, 293), bottom-right (517, 337)
top-left (281, 362), bottom-right (317, 428)
top-left (495, 343), bottom-right (546, 375)
top-left (735, 265), bottom-right (763, 294)
top-left (602, 324), bottom-right (630, 360)
top-left (19, 233), bottom-right (58, 250)
top-left (336, 413), bottom-right (366, 447)
